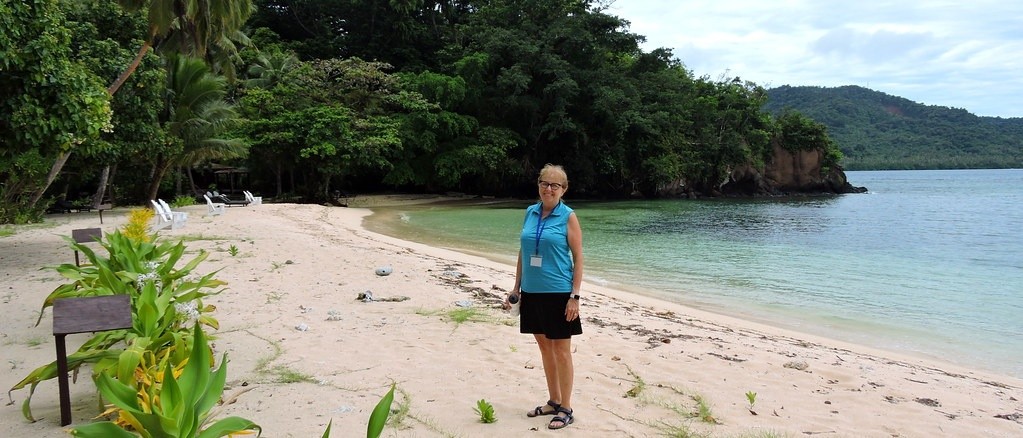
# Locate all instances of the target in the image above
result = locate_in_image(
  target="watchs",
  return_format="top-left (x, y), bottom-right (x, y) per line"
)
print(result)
top-left (570, 293), bottom-right (581, 300)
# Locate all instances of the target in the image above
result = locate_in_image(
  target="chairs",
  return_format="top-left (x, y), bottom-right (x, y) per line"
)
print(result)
top-left (151, 198), bottom-right (188, 234)
top-left (243, 190), bottom-right (263, 207)
top-left (203, 194), bottom-right (226, 218)
top-left (207, 191), bottom-right (250, 207)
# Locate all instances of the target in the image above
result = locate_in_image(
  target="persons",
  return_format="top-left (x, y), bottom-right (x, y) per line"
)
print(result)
top-left (505, 167), bottom-right (584, 430)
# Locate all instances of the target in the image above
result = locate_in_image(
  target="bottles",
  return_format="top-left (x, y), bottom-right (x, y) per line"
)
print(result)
top-left (508, 294), bottom-right (520, 316)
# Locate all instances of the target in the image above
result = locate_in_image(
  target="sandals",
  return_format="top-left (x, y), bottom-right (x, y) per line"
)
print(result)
top-left (548, 407), bottom-right (574, 429)
top-left (526, 399), bottom-right (561, 417)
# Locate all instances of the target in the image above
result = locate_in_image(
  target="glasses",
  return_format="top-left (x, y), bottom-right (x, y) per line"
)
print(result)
top-left (539, 180), bottom-right (564, 191)
